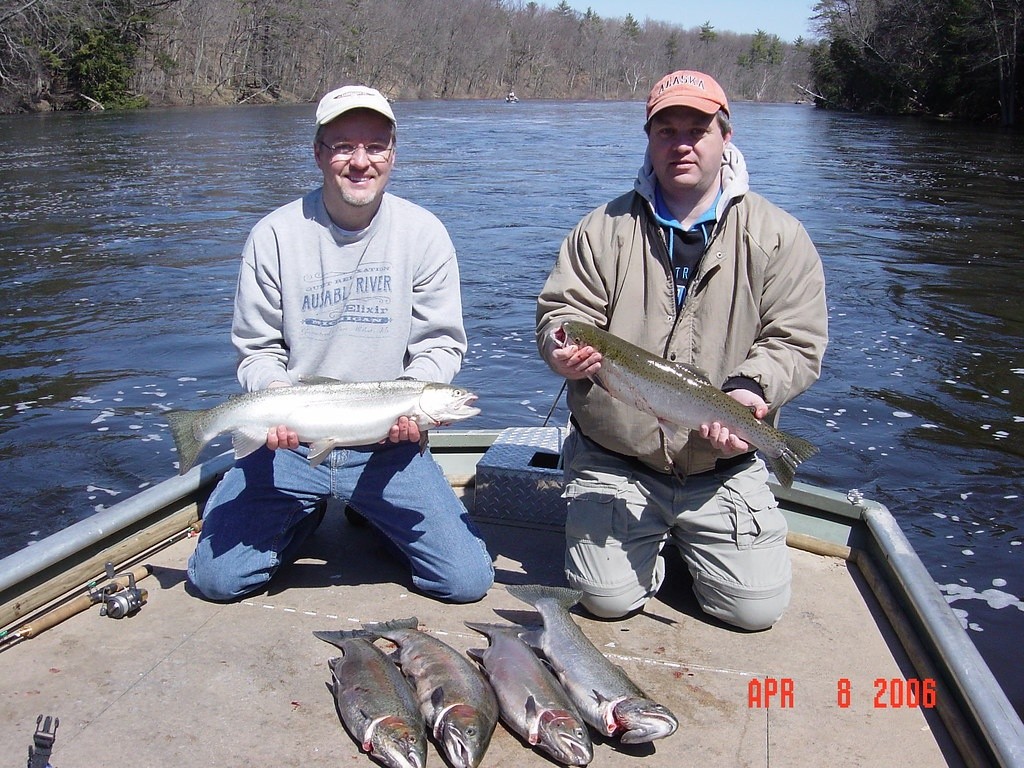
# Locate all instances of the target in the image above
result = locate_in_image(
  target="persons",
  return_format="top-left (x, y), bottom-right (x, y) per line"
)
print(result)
top-left (508, 89), bottom-right (515, 101)
top-left (535, 70), bottom-right (829, 630)
top-left (186, 85), bottom-right (494, 604)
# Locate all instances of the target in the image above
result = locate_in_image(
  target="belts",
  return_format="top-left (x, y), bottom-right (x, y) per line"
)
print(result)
top-left (570, 414), bottom-right (759, 477)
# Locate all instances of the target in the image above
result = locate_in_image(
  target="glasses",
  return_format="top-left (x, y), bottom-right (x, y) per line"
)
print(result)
top-left (320, 139), bottom-right (393, 164)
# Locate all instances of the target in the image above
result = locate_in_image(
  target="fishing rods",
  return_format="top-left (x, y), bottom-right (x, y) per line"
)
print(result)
top-left (0, 562), bottom-right (154, 651)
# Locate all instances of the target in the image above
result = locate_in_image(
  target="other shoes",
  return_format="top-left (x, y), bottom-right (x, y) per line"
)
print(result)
top-left (345, 505), bottom-right (369, 522)
top-left (316, 498), bottom-right (327, 533)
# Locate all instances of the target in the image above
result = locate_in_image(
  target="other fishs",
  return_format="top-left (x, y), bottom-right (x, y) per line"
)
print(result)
top-left (312, 585), bottom-right (677, 768)
top-left (549, 320), bottom-right (820, 491)
top-left (167, 380), bottom-right (482, 475)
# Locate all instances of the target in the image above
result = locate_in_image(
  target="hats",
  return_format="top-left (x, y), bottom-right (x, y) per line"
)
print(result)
top-left (644, 70), bottom-right (730, 122)
top-left (316, 85), bottom-right (396, 137)
